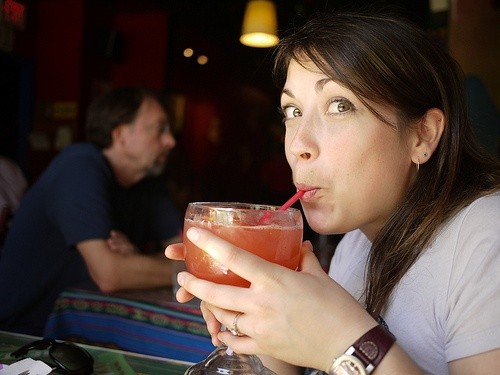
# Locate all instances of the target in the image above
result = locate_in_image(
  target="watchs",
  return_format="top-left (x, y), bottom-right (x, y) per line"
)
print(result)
top-left (327, 324), bottom-right (396, 375)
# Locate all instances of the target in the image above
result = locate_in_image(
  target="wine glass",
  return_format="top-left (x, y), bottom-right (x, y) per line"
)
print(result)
top-left (183, 201), bottom-right (304, 375)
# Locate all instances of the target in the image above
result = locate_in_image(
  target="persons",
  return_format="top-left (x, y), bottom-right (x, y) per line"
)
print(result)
top-left (0, 86), bottom-right (278, 339)
top-left (165, 15), bottom-right (500, 375)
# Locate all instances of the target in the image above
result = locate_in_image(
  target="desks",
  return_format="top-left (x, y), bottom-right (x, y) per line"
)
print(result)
top-left (0, 331), bottom-right (197, 375)
top-left (59, 282), bottom-right (216, 365)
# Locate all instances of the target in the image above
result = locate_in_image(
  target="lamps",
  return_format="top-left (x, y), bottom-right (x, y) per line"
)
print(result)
top-left (239, 0), bottom-right (280, 49)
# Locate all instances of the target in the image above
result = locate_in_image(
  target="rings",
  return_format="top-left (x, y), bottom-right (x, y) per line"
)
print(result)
top-left (229, 312), bottom-right (246, 337)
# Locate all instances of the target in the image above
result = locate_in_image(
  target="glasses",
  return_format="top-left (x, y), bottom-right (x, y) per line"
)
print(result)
top-left (10, 338), bottom-right (95, 375)
